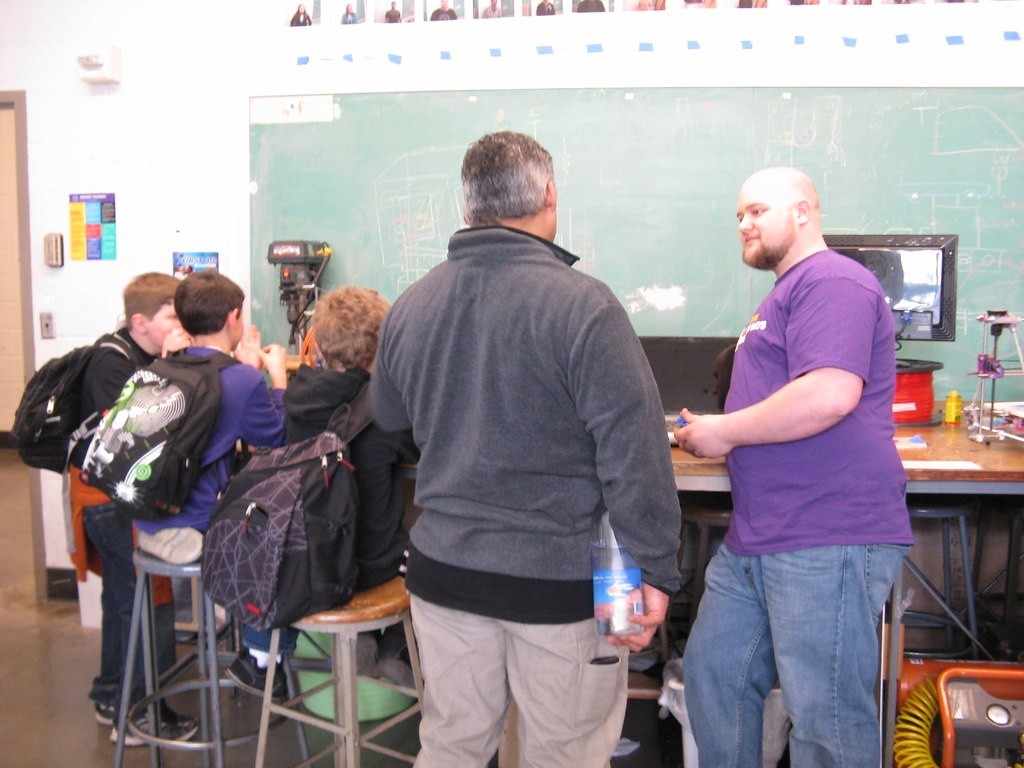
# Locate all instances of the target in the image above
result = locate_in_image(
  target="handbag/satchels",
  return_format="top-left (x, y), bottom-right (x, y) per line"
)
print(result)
top-left (198, 381), bottom-right (380, 634)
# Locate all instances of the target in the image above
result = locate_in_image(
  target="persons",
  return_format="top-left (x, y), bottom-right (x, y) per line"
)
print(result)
top-left (134, 270), bottom-right (299, 705)
top-left (68, 274), bottom-right (198, 746)
top-left (430, 0), bottom-right (457, 21)
top-left (685, 0), bottom-right (717, 8)
top-left (577, 0), bottom-right (605, 12)
top-left (341, 4), bottom-right (356, 24)
top-left (290, 4), bottom-right (311, 26)
top-left (385, 2), bottom-right (401, 24)
top-left (673, 169), bottom-right (913, 768)
top-left (737, 0), bottom-right (767, 9)
top-left (842, 0), bottom-right (872, 5)
top-left (636, 0), bottom-right (666, 11)
top-left (536, 0), bottom-right (555, 16)
top-left (283, 287), bottom-right (425, 692)
top-left (790, 0), bottom-right (820, 6)
top-left (368, 130), bottom-right (682, 768)
top-left (482, 0), bottom-right (501, 18)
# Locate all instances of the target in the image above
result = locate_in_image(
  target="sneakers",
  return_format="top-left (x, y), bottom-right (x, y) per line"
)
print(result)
top-left (109, 706), bottom-right (199, 747)
top-left (223, 646), bottom-right (288, 698)
top-left (95, 689), bottom-right (178, 725)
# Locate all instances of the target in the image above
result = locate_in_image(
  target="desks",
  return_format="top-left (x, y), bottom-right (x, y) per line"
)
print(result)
top-left (396, 399), bottom-right (1024, 496)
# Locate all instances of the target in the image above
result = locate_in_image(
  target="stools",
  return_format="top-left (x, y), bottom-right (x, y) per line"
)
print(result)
top-left (111, 546), bottom-right (425, 768)
top-left (664, 501), bottom-right (732, 654)
top-left (903, 497), bottom-right (980, 663)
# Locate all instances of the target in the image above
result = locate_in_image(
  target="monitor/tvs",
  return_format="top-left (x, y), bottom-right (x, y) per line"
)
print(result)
top-left (637, 336), bottom-right (738, 422)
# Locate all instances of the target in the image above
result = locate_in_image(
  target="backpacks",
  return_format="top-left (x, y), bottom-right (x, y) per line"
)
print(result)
top-left (78, 341), bottom-right (242, 519)
top-left (12, 332), bottom-right (140, 474)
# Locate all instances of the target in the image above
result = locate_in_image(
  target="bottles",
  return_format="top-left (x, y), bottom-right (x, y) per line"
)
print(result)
top-left (592, 499), bottom-right (645, 637)
top-left (945, 390), bottom-right (962, 425)
